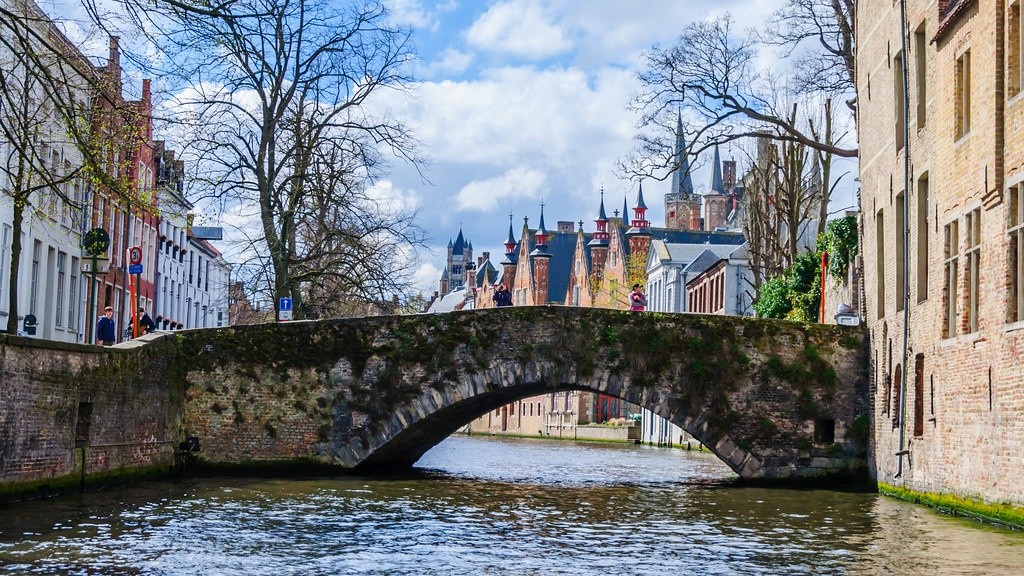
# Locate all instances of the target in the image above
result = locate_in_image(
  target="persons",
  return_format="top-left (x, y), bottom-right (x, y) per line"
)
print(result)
top-left (628, 283), bottom-right (647, 311)
top-left (492, 283), bottom-right (514, 308)
top-left (96, 306), bottom-right (116, 348)
top-left (127, 306), bottom-right (156, 338)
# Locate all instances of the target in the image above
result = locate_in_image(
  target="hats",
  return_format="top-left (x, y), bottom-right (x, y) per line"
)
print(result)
top-left (633, 284), bottom-right (643, 290)
